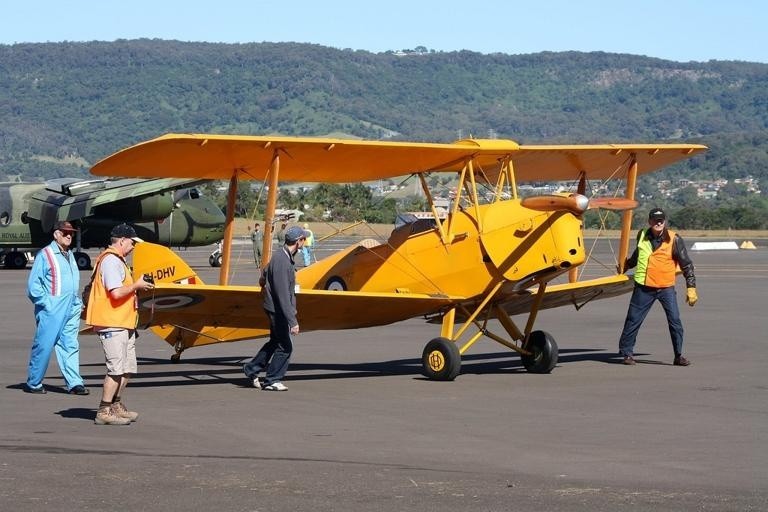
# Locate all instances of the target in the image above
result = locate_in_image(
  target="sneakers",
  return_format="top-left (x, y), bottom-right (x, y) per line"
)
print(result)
top-left (95, 401), bottom-right (138, 426)
top-left (243, 363), bottom-right (289, 391)
top-left (624, 355), bottom-right (635, 365)
top-left (673, 355), bottom-right (690, 366)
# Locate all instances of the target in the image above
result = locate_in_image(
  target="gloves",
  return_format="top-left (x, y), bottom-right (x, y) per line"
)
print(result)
top-left (686, 287), bottom-right (698, 306)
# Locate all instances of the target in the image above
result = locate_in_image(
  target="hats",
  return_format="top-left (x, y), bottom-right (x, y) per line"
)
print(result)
top-left (649, 208), bottom-right (665, 221)
top-left (285, 226), bottom-right (310, 242)
top-left (303, 224), bottom-right (309, 229)
top-left (110, 222), bottom-right (144, 243)
top-left (53, 221), bottom-right (79, 232)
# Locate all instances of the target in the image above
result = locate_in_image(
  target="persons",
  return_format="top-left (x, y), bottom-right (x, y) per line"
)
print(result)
top-left (616, 209), bottom-right (698, 367)
top-left (24, 221), bottom-right (90, 396)
top-left (278, 224), bottom-right (287, 246)
top-left (250, 223), bottom-right (264, 271)
top-left (302, 223), bottom-right (315, 267)
top-left (283, 216), bottom-right (289, 226)
top-left (243, 226), bottom-right (312, 390)
top-left (80, 223), bottom-right (156, 426)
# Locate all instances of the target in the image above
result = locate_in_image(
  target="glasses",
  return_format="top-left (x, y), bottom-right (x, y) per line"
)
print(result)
top-left (58, 232), bottom-right (75, 238)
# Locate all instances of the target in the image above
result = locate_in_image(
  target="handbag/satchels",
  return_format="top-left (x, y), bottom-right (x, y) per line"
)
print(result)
top-left (78, 285), bottom-right (91, 320)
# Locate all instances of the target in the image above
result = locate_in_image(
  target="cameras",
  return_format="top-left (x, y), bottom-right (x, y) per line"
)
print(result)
top-left (144, 272), bottom-right (154, 283)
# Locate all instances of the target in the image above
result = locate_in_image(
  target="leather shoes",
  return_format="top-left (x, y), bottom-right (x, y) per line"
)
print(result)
top-left (67, 384), bottom-right (90, 395)
top-left (25, 383), bottom-right (47, 394)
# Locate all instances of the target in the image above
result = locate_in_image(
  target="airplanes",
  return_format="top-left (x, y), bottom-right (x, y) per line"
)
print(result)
top-left (85, 129), bottom-right (715, 381)
top-left (1, 172), bottom-right (227, 270)
